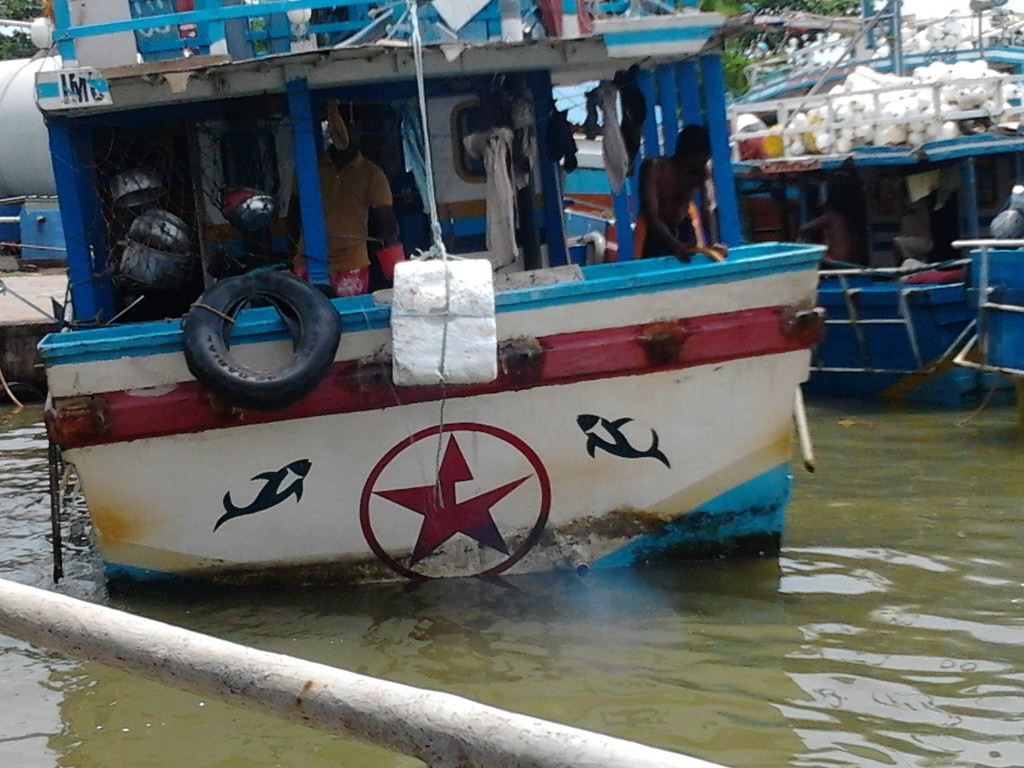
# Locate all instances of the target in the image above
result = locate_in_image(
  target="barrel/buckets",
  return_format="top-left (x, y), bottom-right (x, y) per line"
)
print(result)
top-left (550, 245), bottom-right (586, 267)
top-left (19, 199), bottom-right (68, 258)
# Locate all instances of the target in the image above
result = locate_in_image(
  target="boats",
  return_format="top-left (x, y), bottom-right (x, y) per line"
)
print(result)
top-left (32, 0), bottom-right (828, 595)
top-left (725, 0), bottom-right (1024, 409)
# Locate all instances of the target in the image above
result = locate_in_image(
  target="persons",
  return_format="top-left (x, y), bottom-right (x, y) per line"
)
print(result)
top-left (284, 108), bottom-right (399, 298)
top-left (765, 23), bottom-right (786, 51)
top-left (912, 159), bottom-right (968, 264)
top-left (844, 171), bottom-right (882, 267)
top-left (639, 125), bottom-right (727, 264)
top-left (799, 185), bottom-right (860, 264)
top-left (978, 154), bottom-right (1015, 225)
top-left (850, 167), bottom-right (933, 267)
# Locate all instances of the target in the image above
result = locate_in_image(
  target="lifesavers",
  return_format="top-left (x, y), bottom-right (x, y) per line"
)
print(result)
top-left (182, 268), bottom-right (344, 406)
